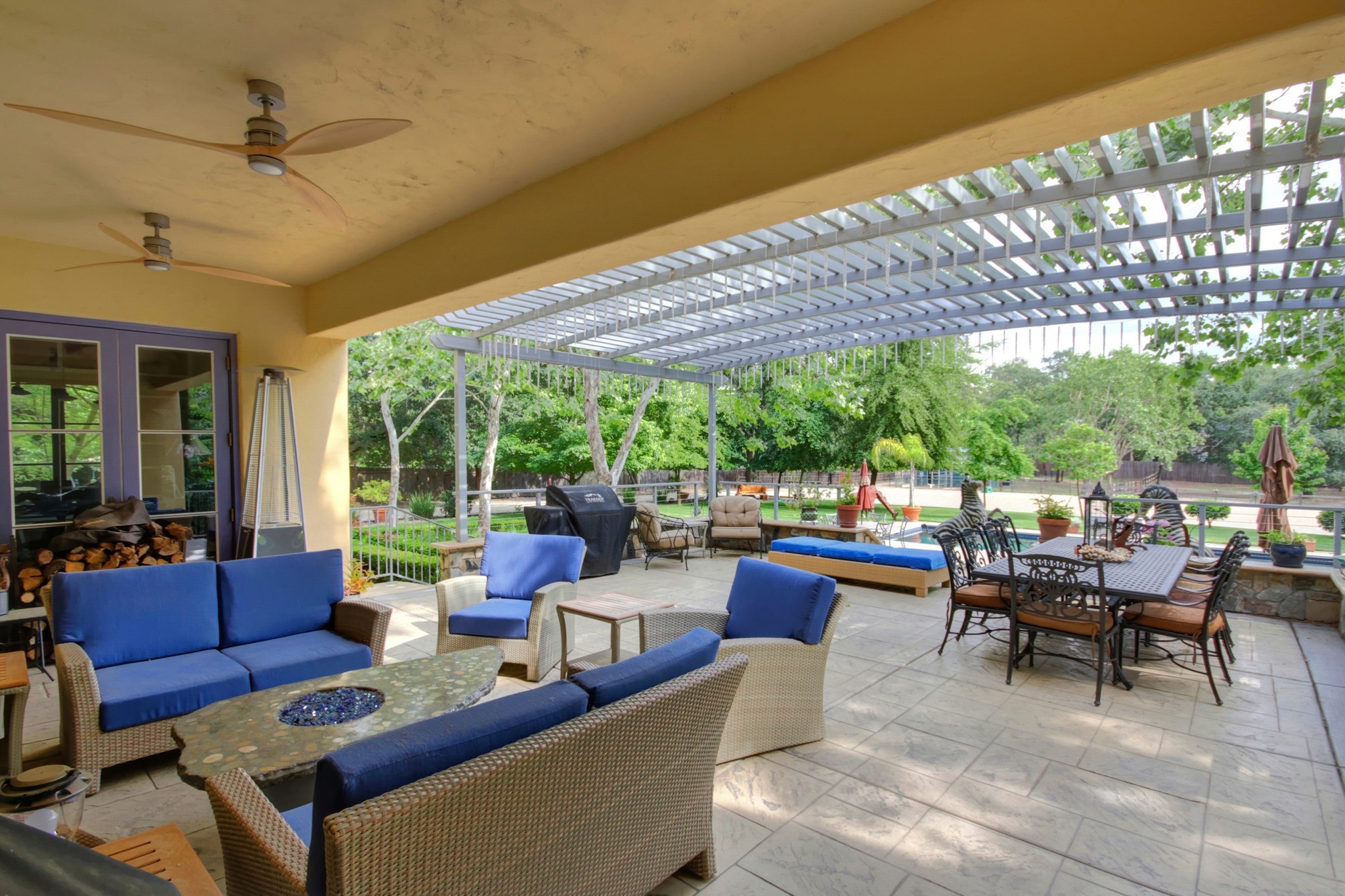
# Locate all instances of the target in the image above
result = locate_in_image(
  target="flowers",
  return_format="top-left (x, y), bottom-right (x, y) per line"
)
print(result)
top-left (836, 465), bottom-right (858, 505)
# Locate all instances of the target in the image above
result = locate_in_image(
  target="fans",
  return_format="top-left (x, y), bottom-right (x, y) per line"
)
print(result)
top-left (54, 212), bottom-right (292, 288)
top-left (3, 78), bottom-right (413, 233)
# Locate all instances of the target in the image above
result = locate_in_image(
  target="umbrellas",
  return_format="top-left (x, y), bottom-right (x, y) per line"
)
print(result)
top-left (1255, 422), bottom-right (1298, 554)
top-left (854, 458), bottom-right (874, 525)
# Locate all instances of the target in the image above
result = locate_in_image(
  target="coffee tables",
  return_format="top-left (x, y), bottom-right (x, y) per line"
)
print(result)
top-left (171, 645), bottom-right (504, 813)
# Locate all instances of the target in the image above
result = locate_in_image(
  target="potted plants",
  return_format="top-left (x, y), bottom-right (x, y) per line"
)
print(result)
top-left (1031, 492), bottom-right (1075, 543)
top-left (1259, 528), bottom-right (1306, 568)
top-left (784, 476), bottom-right (833, 520)
top-left (901, 505), bottom-right (922, 521)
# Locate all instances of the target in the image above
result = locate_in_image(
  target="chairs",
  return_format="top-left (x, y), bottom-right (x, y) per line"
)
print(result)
top-left (434, 497), bottom-right (1251, 896)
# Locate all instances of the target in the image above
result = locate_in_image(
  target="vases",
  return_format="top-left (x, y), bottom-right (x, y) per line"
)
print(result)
top-left (837, 505), bottom-right (862, 528)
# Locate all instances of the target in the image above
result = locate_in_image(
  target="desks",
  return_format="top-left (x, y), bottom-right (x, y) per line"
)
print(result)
top-left (856, 521), bottom-right (891, 535)
top-left (556, 593), bottom-right (674, 681)
top-left (661, 520), bottom-right (708, 561)
top-left (972, 536), bottom-right (1193, 691)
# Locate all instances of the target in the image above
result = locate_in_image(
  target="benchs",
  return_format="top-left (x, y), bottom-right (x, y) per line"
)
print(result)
top-left (41, 548), bottom-right (390, 793)
top-left (206, 627), bottom-right (748, 895)
top-left (768, 536), bottom-right (951, 597)
top-left (735, 484), bottom-right (769, 500)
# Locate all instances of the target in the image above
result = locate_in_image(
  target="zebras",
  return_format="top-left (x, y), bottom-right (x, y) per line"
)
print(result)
top-left (1137, 484), bottom-right (1216, 557)
top-left (921, 478), bottom-right (1006, 571)
